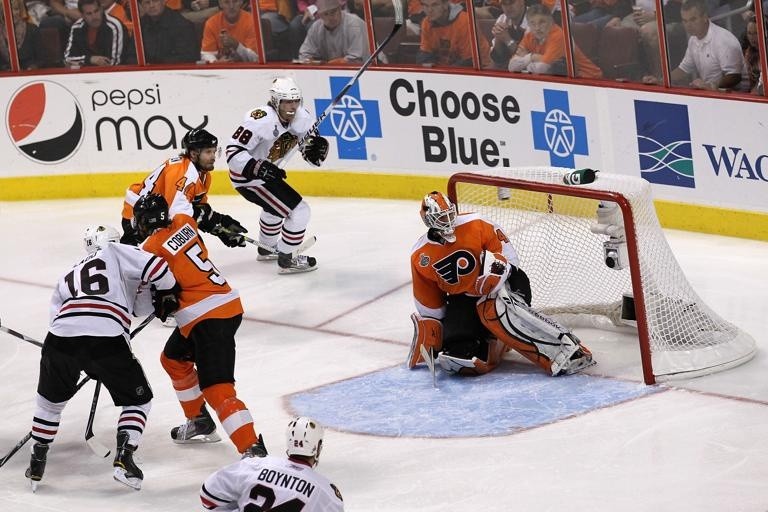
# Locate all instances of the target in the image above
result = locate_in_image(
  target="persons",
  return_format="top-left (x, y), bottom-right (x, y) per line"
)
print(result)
top-left (569, 1), bottom-right (635, 59)
top-left (200, 1), bottom-right (260, 63)
top-left (181, 1), bottom-right (219, 12)
top-left (119, 129), bottom-right (248, 326)
top-left (62, 1), bottom-right (132, 67)
top-left (298, 0), bottom-right (365, 64)
top-left (642, 2), bottom-right (750, 90)
top-left (407, 192), bottom-right (596, 376)
top-left (127, 0), bottom-right (201, 65)
top-left (23, 1), bottom-right (80, 26)
top-left (226, 79), bottom-right (329, 274)
top-left (632, 1), bottom-right (687, 74)
top-left (719, 1), bottom-right (756, 50)
top-left (98, 0), bottom-right (132, 37)
top-left (737, 16), bottom-right (768, 95)
top-left (508, 4), bottom-right (604, 78)
top-left (287, 1), bottom-right (347, 59)
top-left (488, 0), bottom-right (531, 70)
top-left (25, 223), bottom-right (181, 492)
top-left (416, 0), bottom-right (494, 70)
top-left (200, 416), bottom-right (344, 512)
top-left (134, 194), bottom-right (268, 459)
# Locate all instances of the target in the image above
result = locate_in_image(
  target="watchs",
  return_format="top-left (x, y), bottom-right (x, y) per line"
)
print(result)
top-left (507, 40), bottom-right (514, 49)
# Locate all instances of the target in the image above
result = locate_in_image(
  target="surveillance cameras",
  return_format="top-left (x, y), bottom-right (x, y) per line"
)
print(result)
top-left (603, 239), bottom-right (629, 271)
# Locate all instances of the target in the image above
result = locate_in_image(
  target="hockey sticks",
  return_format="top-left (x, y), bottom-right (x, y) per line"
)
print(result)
top-left (276, 0), bottom-right (403, 169)
top-left (221, 227), bottom-right (316, 255)
top-left (83, 381), bottom-right (111, 459)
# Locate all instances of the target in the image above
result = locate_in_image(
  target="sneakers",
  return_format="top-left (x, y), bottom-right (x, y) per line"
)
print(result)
top-left (258, 246), bottom-right (278, 255)
top-left (25, 445), bottom-right (49, 481)
top-left (114, 443), bottom-right (143, 480)
top-left (171, 411), bottom-right (216, 440)
top-left (561, 344), bottom-right (591, 371)
top-left (241, 439), bottom-right (266, 463)
top-left (278, 253), bottom-right (316, 268)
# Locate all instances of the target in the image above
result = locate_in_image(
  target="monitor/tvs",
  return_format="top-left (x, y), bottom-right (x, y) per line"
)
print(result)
top-left (619, 295), bottom-right (638, 328)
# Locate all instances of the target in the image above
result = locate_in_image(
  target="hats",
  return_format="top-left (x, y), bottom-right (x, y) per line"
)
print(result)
top-left (287, 416), bottom-right (325, 460)
top-left (317, 0), bottom-right (339, 13)
top-left (134, 194), bottom-right (168, 227)
top-left (85, 225), bottom-right (120, 252)
top-left (270, 79), bottom-right (302, 118)
top-left (421, 192), bottom-right (458, 233)
top-left (182, 130), bottom-right (218, 149)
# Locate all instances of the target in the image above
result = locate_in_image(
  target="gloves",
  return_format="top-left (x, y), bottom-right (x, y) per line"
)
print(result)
top-left (217, 218), bottom-right (247, 247)
top-left (476, 261), bottom-right (509, 294)
top-left (307, 137), bottom-right (328, 167)
top-left (155, 289), bottom-right (177, 321)
top-left (257, 162), bottom-right (287, 181)
top-left (422, 319), bottom-right (442, 360)
